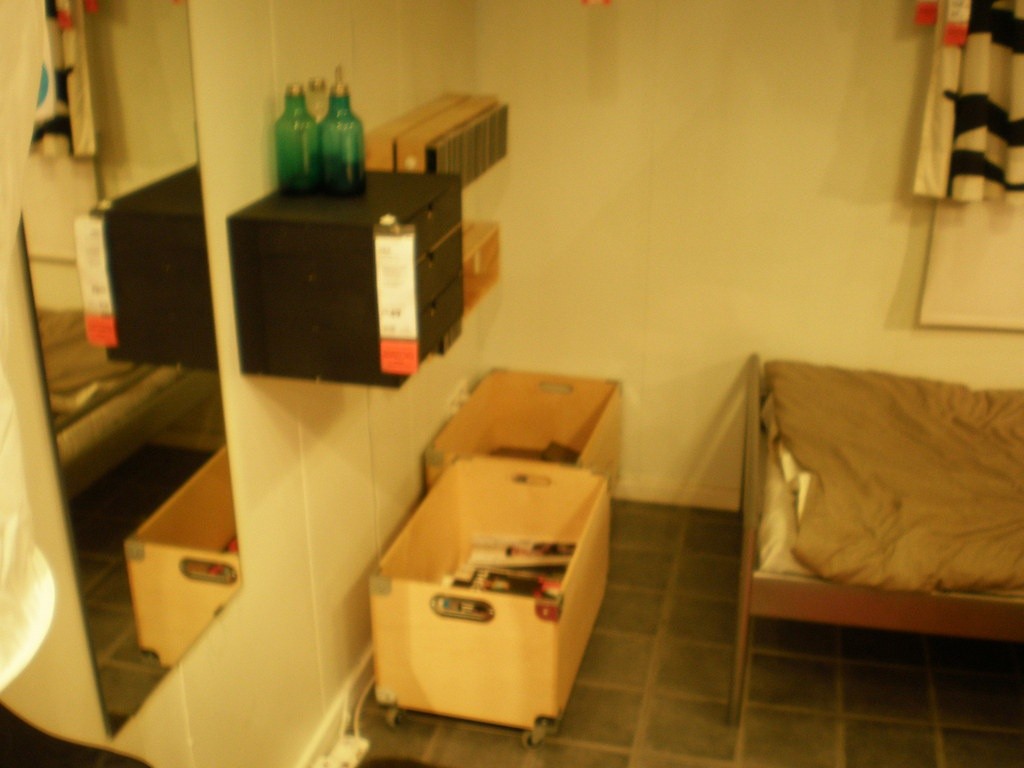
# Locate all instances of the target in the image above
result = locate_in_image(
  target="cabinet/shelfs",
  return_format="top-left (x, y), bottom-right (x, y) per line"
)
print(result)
top-left (74, 158), bottom-right (215, 373)
top-left (227, 169), bottom-right (466, 390)
top-left (363, 91), bottom-right (510, 314)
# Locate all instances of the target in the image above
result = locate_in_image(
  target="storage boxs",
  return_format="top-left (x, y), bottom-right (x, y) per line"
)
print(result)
top-left (368, 453), bottom-right (610, 746)
top-left (424, 366), bottom-right (622, 501)
top-left (124, 443), bottom-right (244, 668)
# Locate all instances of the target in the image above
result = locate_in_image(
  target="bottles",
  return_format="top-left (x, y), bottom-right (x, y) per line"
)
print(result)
top-left (307, 73), bottom-right (328, 125)
top-left (271, 80), bottom-right (319, 199)
top-left (319, 62), bottom-right (369, 200)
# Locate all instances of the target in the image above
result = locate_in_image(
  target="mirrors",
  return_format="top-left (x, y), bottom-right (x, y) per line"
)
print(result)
top-left (21, 0), bottom-right (244, 740)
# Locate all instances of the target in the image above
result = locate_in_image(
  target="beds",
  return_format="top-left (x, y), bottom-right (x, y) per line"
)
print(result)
top-left (36, 306), bottom-right (196, 504)
top-left (726, 351), bottom-right (1024, 725)
